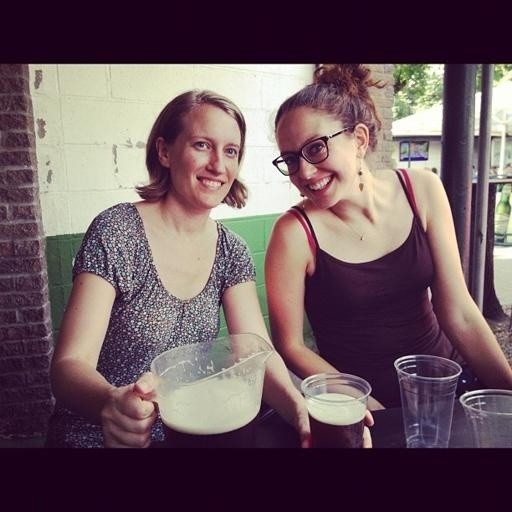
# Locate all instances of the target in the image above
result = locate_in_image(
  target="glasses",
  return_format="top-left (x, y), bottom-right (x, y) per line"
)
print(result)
top-left (272, 126), bottom-right (355, 176)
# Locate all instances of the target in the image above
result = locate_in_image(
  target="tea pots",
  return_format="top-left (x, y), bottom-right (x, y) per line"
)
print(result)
top-left (143, 332), bottom-right (273, 448)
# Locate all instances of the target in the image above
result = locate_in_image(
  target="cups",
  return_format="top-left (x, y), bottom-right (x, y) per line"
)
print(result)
top-left (299, 371), bottom-right (372, 448)
top-left (459, 388), bottom-right (511, 448)
top-left (392, 354), bottom-right (463, 448)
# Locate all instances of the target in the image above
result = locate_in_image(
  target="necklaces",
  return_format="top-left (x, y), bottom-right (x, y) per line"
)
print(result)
top-left (337, 185), bottom-right (375, 240)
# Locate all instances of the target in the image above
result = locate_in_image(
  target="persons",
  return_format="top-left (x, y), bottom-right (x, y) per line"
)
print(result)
top-left (45, 89), bottom-right (375, 449)
top-left (265, 64), bottom-right (512, 413)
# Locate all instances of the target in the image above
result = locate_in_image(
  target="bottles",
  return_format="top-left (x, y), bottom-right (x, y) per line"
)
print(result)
top-left (494, 182), bottom-right (512, 242)
